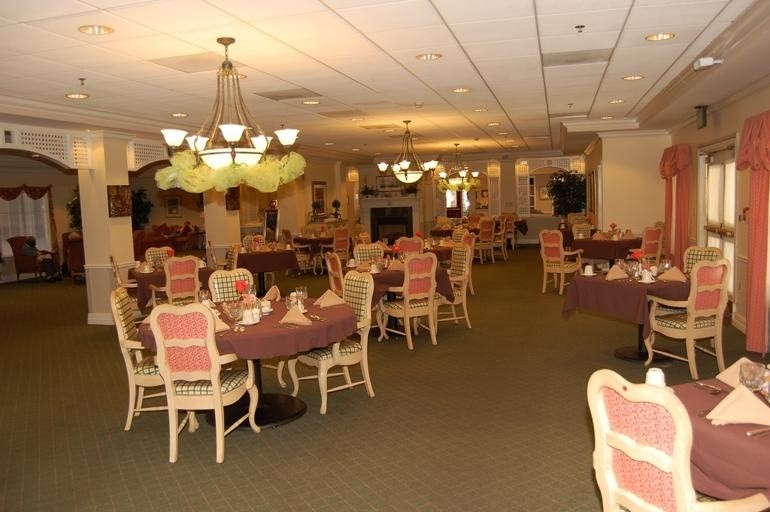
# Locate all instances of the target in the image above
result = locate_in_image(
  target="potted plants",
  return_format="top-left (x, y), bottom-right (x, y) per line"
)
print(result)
top-left (404, 183), bottom-right (416, 198)
top-left (359, 184), bottom-right (374, 198)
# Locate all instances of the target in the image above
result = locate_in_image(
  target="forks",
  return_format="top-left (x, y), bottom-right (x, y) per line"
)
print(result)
top-left (694, 384), bottom-right (720, 394)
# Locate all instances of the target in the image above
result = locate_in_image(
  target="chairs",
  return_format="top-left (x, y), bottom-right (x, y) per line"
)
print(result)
top-left (294, 210), bottom-right (517, 351)
top-left (7, 224), bottom-right (198, 284)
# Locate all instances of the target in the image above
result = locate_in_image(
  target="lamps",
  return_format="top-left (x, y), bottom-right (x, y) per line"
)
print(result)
top-left (161, 38), bottom-right (299, 171)
top-left (377, 120), bottom-right (438, 183)
top-left (438, 144), bottom-right (479, 186)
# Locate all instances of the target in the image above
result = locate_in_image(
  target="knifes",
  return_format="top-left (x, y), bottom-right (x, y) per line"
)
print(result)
top-left (695, 381), bottom-right (730, 394)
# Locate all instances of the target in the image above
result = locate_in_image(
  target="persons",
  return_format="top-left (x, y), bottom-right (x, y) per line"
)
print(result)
top-left (162, 221), bottom-right (191, 239)
top-left (62, 232), bottom-right (80, 282)
top-left (21, 237), bottom-right (62, 283)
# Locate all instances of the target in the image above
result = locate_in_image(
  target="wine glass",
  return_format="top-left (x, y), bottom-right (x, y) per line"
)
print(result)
top-left (196, 284), bottom-right (308, 333)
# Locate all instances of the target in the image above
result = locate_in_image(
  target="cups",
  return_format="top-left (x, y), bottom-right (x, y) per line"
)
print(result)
top-left (370, 253), bottom-right (406, 266)
top-left (644, 368), bottom-right (666, 387)
top-left (370, 265), bottom-right (377, 272)
top-left (349, 259), bottom-right (355, 266)
top-left (740, 363), bottom-right (766, 392)
top-left (585, 258), bottom-right (672, 281)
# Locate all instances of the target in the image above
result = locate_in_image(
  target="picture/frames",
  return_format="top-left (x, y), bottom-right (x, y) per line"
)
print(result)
top-left (312, 182), bottom-right (328, 217)
top-left (165, 195), bottom-right (182, 218)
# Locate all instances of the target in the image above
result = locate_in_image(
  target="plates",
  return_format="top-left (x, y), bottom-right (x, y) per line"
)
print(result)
top-left (581, 274), bottom-right (597, 277)
top-left (369, 271), bottom-right (380, 274)
top-left (347, 265), bottom-right (357, 268)
top-left (140, 271), bottom-right (153, 274)
top-left (639, 281), bottom-right (655, 284)
